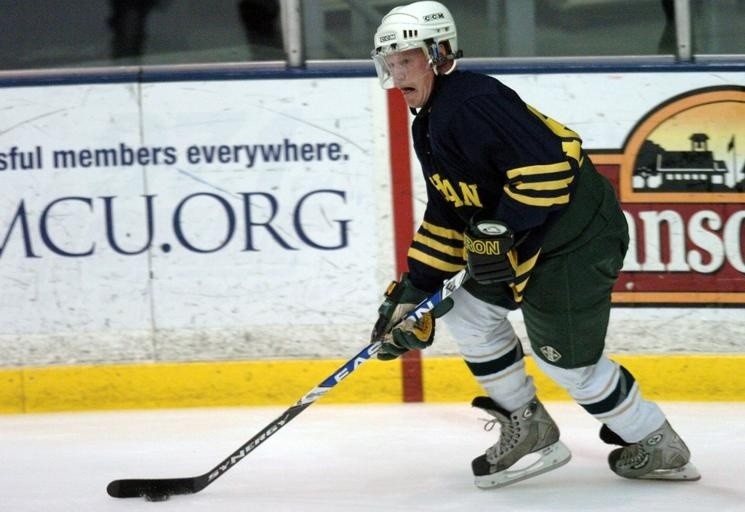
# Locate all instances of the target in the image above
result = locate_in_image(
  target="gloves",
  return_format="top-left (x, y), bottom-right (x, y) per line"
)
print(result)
top-left (371, 271), bottom-right (453, 360)
top-left (463, 217), bottom-right (518, 284)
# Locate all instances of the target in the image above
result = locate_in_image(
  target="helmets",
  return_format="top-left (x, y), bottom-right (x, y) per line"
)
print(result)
top-left (370, 0), bottom-right (458, 90)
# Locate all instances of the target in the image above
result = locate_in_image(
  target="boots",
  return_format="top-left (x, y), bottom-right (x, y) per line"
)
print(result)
top-left (472, 394), bottom-right (560, 475)
top-left (599, 422), bottom-right (691, 478)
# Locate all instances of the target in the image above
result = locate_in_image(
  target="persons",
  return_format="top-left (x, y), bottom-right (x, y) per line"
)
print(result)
top-left (369, 1), bottom-right (700, 478)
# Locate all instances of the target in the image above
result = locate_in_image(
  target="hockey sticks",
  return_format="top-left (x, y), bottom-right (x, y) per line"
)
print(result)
top-left (107, 266), bottom-right (470, 497)
